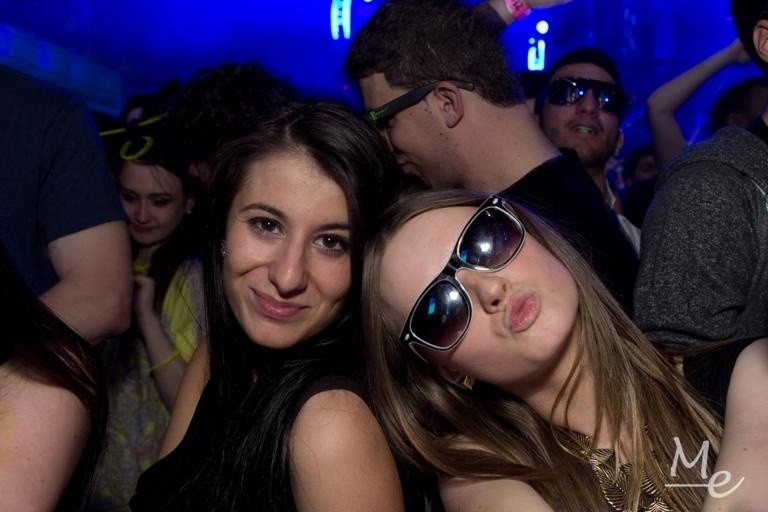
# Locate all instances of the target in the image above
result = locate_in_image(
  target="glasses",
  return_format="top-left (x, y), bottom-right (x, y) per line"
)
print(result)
top-left (540, 77), bottom-right (630, 116)
top-left (400, 193), bottom-right (527, 367)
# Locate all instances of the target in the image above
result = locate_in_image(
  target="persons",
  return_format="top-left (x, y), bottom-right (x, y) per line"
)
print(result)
top-left (0, 0), bottom-right (768, 512)
